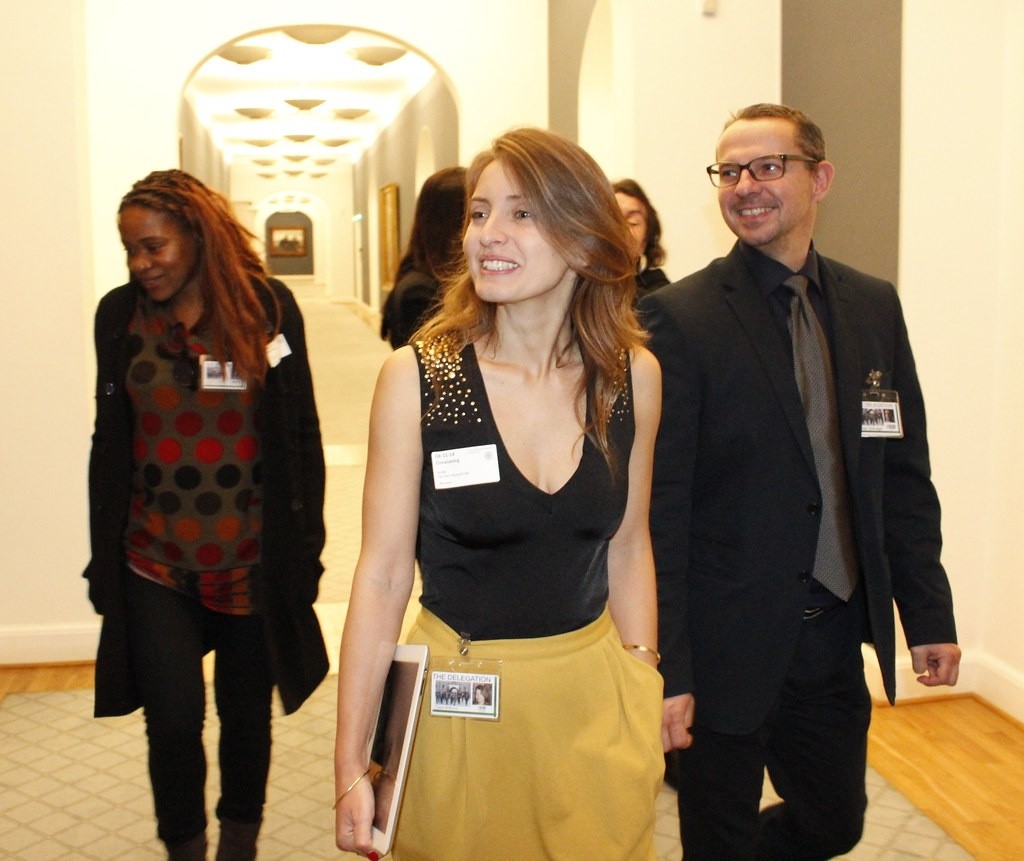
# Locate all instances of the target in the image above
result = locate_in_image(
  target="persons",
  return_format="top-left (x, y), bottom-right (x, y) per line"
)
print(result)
top-left (650, 101), bottom-right (962, 861)
top-left (473, 685), bottom-right (491, 705)
top-left (332, 127), bottom-right (666, 860)
top-left (436, 689), bottom-right (469, 706)
top-left (862, 412), bottom-right (883, 425)
top-left (884, 409), bottom-right (894, 422)
top-left (80, 168), bottom-right (331, 860)
top-left (378, 168), bottom-right (476, 350)
top-left (603, 180), bottom-right (673, 299)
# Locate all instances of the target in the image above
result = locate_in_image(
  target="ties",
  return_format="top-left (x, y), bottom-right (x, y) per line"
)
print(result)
top-left (781, 274), bottom-right (863, 602)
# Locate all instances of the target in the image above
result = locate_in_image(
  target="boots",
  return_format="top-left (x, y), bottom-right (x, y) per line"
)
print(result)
top-left (162, 831), bottom-right (205, 860)
top-left (215, 820), bottom-right (260, 859)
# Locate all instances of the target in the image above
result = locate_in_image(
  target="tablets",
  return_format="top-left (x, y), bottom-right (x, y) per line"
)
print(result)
top-left (368, 643), bottom-right (429, 853)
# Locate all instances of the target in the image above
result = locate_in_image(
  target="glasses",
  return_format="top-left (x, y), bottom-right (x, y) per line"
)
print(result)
top-left (705, 153), bottom-right (819, 189)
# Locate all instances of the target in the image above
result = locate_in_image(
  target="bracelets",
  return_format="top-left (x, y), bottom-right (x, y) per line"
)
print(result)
top-left (332, 766), bottom-right (369, 810)
top-left (621, 642), bottom-right (660, 670)
